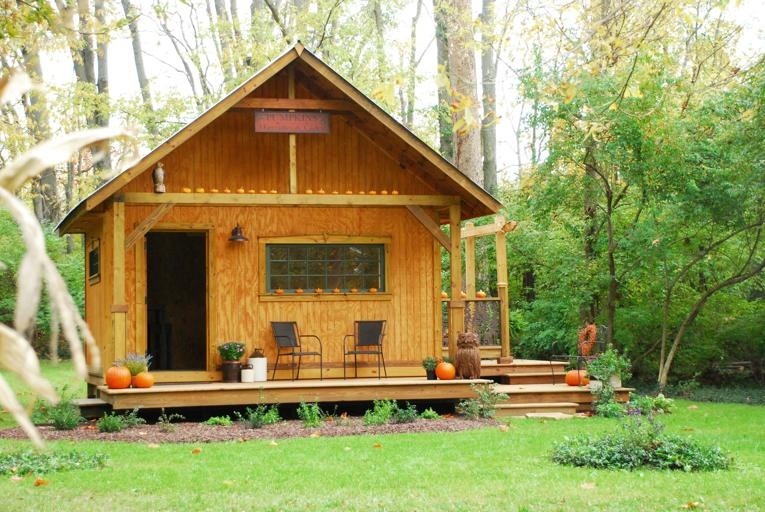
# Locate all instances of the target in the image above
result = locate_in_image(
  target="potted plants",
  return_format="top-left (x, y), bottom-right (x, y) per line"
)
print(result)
top-left (586, 341), bottom-right (633, 388)
top-left (422, 356), bottom-right (439, 380)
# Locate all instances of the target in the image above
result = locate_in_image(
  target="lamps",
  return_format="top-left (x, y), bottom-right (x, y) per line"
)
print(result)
top-left (228, 222), bottom-right (248, 242)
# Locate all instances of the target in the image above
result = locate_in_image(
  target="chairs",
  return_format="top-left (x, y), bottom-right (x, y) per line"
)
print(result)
top-left (343, 320), bottom-right (388, 380)
top-left (550, 325), bottom-right (609, 388)
top-left (270, 321), bottom-right (323, 381)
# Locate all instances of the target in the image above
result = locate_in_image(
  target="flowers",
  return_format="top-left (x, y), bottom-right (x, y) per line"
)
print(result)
top-left (218, 342), bottom-right (246, 361)
top-left (112, 352), bottom-right (155, 375)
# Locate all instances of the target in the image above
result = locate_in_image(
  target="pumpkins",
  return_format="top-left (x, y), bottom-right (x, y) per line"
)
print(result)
top-left (435, 362), bottom-right (455, 380)
top-left (276, 288), bottom-right (377, 294)
top-left (106, 366), bottom-right (131, 389)
top-left (136, 372), bottom-right (153, 388)
top-left (441, 290), bottom-right (486, 299)
top-left (565, 370), bottom-right (589, 386)
top-left (183, 186), bottom-right (277, 194)
top-left (305, 188), bottom-right (398, 195)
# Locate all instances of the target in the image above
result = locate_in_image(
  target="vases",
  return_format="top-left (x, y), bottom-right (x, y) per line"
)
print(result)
top-left (130, 374), bottom-right (140, 387)
top-left (221, 360), bottom-right (242, 383)
